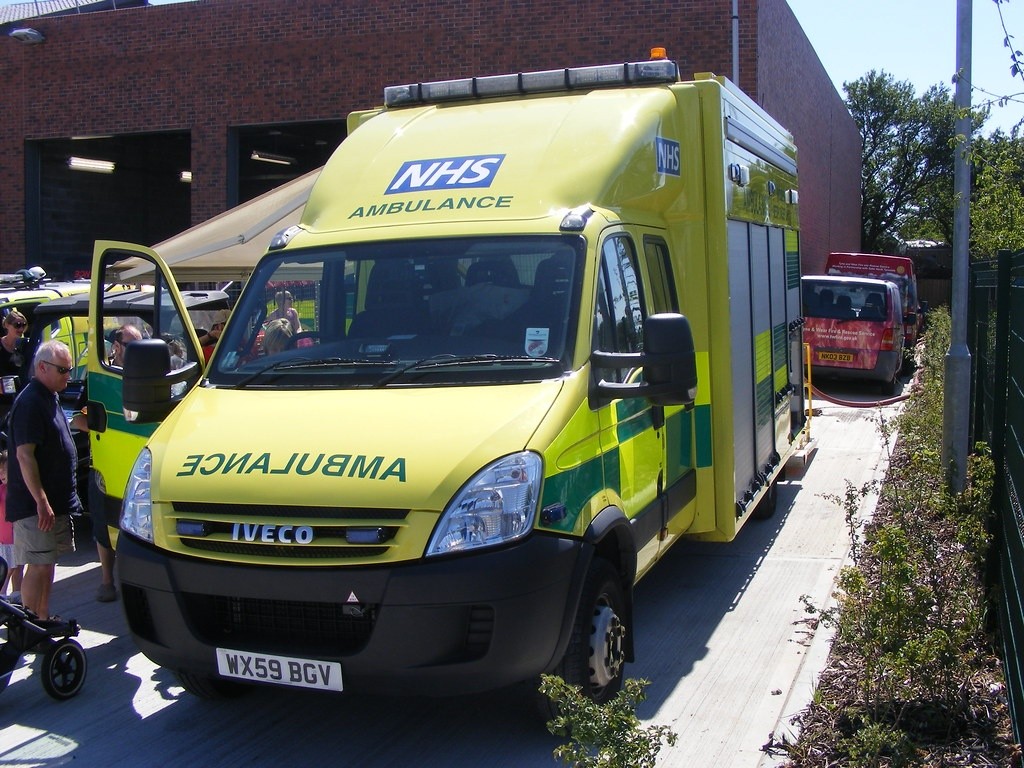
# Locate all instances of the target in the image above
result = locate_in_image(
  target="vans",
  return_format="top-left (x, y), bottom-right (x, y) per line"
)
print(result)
top-left (799, 275), bottom-right (916, 396)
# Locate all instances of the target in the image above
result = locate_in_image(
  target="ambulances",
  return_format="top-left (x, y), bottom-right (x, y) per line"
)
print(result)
top-left (88, 48), bottom-right (809, 712)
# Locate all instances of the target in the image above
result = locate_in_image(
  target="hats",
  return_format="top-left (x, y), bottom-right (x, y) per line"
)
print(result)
top-left (210, 309), bottom-right (232, 332)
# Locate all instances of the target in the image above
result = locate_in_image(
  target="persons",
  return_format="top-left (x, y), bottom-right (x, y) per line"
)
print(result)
top-left (0, 450), bottom-right (25, 607)
top-left (0, 312), bottom-right (35, 446)
top-left (72, 325), bottom-right (143, 602)
top-left (160, 291), bottom-right (313, 397)
top-left (5, 339), bottom-right (83, 632)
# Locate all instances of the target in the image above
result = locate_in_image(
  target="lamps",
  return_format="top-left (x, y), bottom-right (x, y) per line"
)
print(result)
top-left (250, 150), bottom-right (296, 165)
top-left (8, 28), bottom-right (45, 45)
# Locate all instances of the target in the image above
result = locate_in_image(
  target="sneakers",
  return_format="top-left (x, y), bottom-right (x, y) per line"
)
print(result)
top-left (96, 583), bottom-right (117, 602)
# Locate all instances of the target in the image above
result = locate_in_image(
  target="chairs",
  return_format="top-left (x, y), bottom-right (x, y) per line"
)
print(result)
top-left (463, 254), bottom-right (519, 288)
top-left (519, 259), bottom-right (572, 346)
top-left (834, 295), bottom-right (856, 318)
top-left (347, 259), bottom-right (436, 340)
top-left (859, 293), bottom-right (884, 321)
top-left (816, 289), bottom-right (834, 317)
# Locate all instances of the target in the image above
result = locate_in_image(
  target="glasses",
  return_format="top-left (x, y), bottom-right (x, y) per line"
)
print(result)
top-left (282, 297), bottom-right (291, 300)
top-left (6, 321), bottom-right (27, 329)
top-left (40, 360), bottom-right (74, 374)
top-left (115, 338), bottom-right (128, 347)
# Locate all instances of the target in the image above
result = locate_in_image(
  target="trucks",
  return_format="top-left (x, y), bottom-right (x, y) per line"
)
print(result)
top-left (818, 252), bottom-right (923, 376)
top-left (0, 274), bottom-right (165, 380)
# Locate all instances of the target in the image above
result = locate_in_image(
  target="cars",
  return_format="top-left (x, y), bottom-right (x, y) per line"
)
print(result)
top-left (15, 291), bottom-right (233, 465)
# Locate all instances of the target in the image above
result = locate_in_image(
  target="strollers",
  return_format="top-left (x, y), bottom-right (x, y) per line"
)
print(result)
top-left (0, 555), bottom-right (88, 700)
